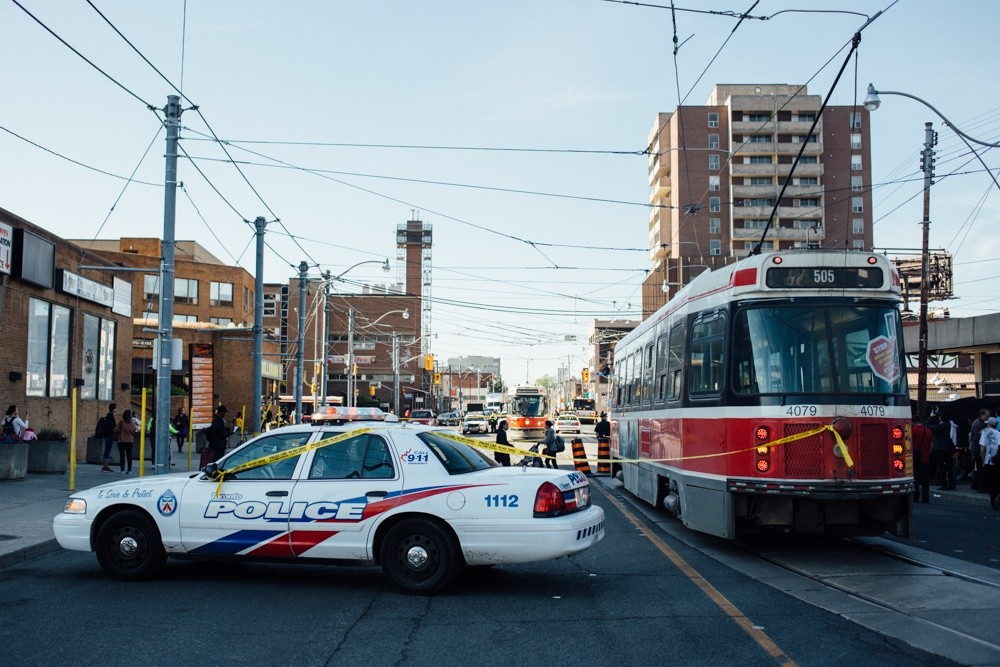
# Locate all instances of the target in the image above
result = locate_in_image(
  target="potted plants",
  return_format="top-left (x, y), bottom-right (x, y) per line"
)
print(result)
top-left (86, 435), bottom-right (121, 466)
top-left (26, 424), bottom-right (69, 474)
top-left (0, 433), bottom-right (30, 479)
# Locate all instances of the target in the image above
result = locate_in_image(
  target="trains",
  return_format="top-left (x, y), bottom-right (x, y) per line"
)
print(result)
top-left (612, 249), bottom-right (920, 544)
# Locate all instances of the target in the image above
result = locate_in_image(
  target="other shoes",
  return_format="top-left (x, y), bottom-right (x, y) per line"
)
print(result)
top-left (915, 484), bottom-right (1000, 511)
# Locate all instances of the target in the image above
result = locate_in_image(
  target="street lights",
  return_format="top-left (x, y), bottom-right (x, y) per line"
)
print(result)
top-left (389, 331), bottom-right (497, 421)
top-left (290, 257), bottom-right (392, 426)
top-left (862, 83), bottom-right (1000, 189)
top-left (345, 307), bottom-right (412, 407)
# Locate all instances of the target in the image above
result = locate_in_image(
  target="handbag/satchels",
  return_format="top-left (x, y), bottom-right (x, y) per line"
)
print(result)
top-left (950, 439), bottom-right (955, 454)
top-left (912, 449), bottom-right (922, 469)
top-left (112, 421), bottom-right (122, 441)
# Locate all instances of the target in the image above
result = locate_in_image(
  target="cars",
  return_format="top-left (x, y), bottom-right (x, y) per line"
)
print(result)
top-left (464, 414), bottom-right (489, 435)
top-left (555, 415), bottom-right (581, 434)
top-left (53, 406), bottom-right (607, 597)
top-left (408, 401), bottom-right (507, 426)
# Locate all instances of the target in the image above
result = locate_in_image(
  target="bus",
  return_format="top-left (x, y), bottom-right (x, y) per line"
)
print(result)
top-left (506, 387), bottom-right (551, 440)
top-left (572, 398), bottom-right (596, 424)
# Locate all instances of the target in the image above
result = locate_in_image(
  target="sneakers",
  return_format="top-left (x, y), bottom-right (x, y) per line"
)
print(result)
top-left (101, 447), bottom-right (183, 474)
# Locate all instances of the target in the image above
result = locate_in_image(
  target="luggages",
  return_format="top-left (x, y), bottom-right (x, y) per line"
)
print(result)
top-left (200, 447), bottom-right (213, 471)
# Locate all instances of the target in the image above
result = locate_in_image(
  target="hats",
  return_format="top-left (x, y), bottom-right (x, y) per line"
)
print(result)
top-left (217, 405), bottom-right (230, 412)
top-left (600, 413), bottom-right (608, 418)
top-left (984, 417), bottom-right (998, 426)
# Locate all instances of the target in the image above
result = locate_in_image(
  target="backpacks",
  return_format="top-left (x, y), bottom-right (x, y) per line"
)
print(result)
top-left (3, 417), bottom-right (18, 439)
top-left (494, 442), bottom-right (502, 463)
top-left (95, 416), bottom-right (106, 439)
top-left (555, 436), bottom-right (565, 452)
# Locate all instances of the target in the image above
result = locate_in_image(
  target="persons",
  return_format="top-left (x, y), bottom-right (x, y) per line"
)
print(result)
top-left (488, 411), bottom-right (498, 433)
top-left (995, 408), bottom-right (1000, 432)
top-left (971, 409), bottom-right (991, 493)
top-left (113, 410), bottom-right (136, 474)
top-left (0, 405), bottom-right (29, 444)
top-left (148, 408), bottom-right (180, 470)
top-left (336, 438), bottom-right (390, 478)
top-left (912, 415), bottom-right (973, 503)
top-left (494, 420), bottom-right (514, 465)
top-left (213, 408), bottom-right (312, 448)
top-left (101, 403), bottom-right (119, 472)
top-left (978, 417), bottom-right (1000, 511)
top-left (536, 421), bottom-right (558, 469)
top-left (132, 411), bottom-right (141, 433)
top-left (209, 405), bottom-right (239, 463)
top-left (594, 414), bottom-right (610, 438)
top-left (174, 406), bottom-right (189, 454)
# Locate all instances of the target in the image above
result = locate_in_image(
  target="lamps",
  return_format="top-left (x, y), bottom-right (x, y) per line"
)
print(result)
top-left (926, 372), bottom-right (962, 400)
